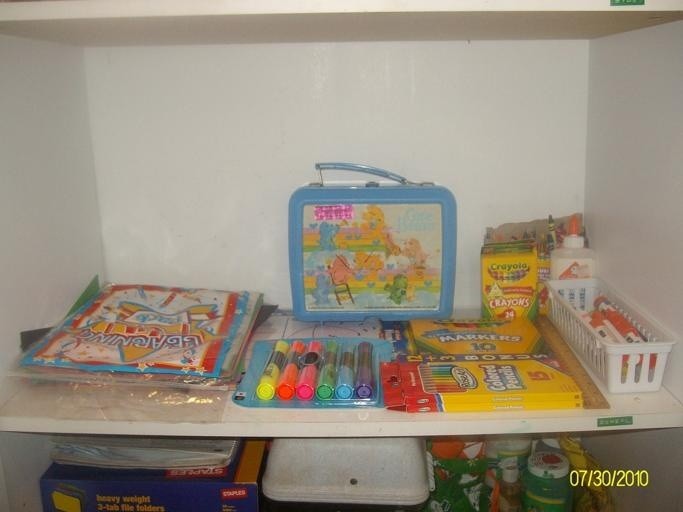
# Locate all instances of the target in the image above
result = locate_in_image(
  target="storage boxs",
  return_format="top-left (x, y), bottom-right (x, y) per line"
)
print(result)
top-left (38, 460), bottom-right (258, 511)
top-left (260, 438), bottom-right (435, 510)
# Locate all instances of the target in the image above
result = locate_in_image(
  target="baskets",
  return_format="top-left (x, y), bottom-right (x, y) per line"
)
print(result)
top-left (543, 277), bottom-right (676, 394)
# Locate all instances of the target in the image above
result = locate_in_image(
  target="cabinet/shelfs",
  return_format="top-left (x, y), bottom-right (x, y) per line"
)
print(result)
top-left (1, 0), bottom-right (683, 512)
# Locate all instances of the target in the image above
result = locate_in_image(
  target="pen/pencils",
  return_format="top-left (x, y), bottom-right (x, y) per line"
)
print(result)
top-left (589, 294), bottom-right (657, 375)
top-left (513, 213), bottom-right (565, 259)
top-left (418, 366), bottom-right (467, 393)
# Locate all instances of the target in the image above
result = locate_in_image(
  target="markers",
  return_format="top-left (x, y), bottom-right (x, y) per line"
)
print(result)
top-left (257, 339), bottom-right (372, 401)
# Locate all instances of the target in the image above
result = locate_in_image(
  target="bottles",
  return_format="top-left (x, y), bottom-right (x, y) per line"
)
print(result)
top-left (482, 434), bottom-right (576, 512)
